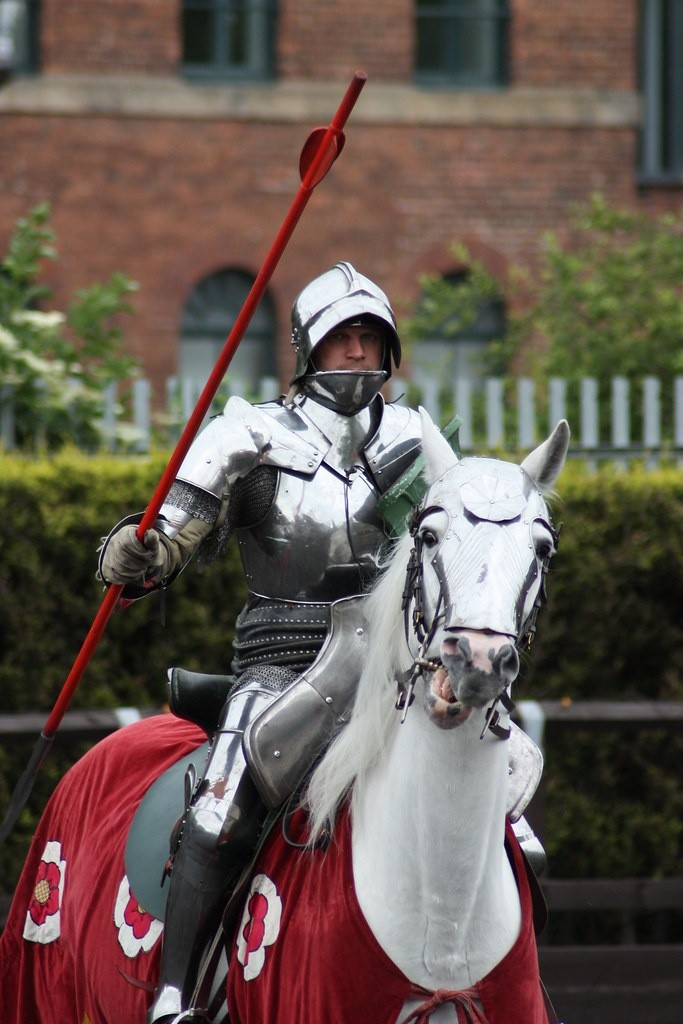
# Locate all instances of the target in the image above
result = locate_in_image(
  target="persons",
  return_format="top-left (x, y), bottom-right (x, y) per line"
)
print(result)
top-left (96, 258), bottom-right (547, 1024)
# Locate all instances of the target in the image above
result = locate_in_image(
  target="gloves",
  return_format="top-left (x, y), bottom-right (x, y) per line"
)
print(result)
top-left (102, 524), bottom-right (166, 585)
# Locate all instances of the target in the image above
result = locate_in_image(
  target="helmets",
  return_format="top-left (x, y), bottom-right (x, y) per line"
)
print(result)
top-left (290, 259), bottom-right (402, 385)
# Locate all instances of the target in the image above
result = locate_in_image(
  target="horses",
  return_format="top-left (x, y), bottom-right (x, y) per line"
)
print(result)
top-left (17, 403), bottom-right (573, 1024)
